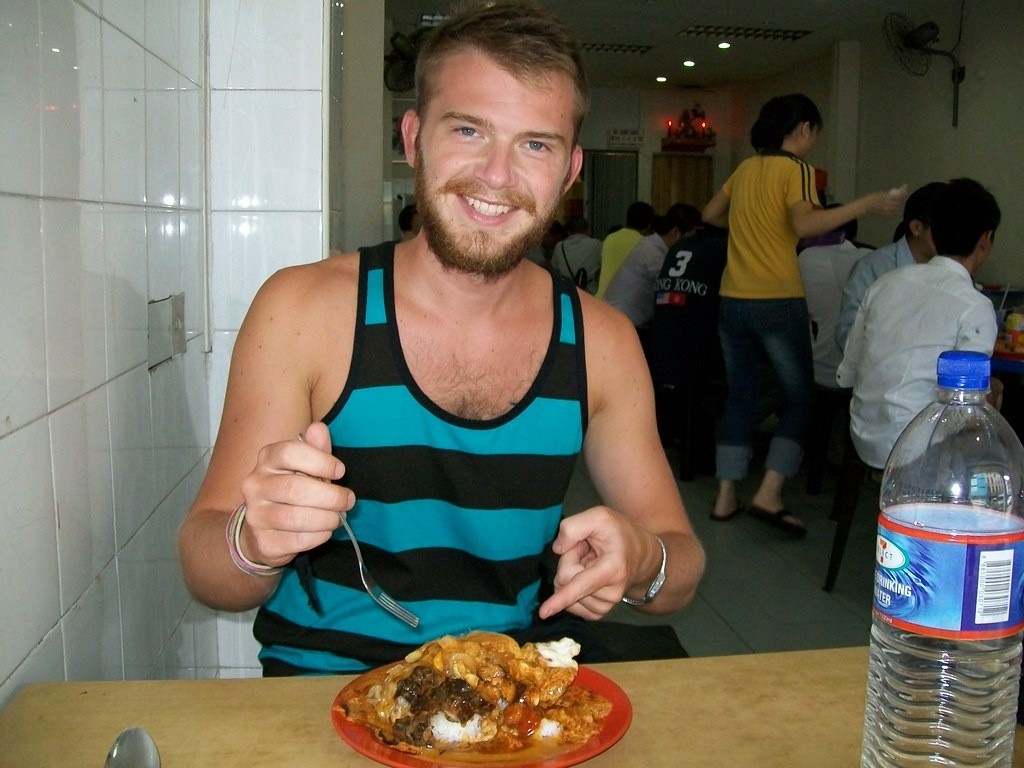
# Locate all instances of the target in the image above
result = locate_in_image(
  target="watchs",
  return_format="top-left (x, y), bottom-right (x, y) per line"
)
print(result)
top-left (620, 536), bottom-right (667, 606)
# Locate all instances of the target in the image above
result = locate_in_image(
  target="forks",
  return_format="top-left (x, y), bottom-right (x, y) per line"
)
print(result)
top-left (297, 434), bottom-right (420, 629)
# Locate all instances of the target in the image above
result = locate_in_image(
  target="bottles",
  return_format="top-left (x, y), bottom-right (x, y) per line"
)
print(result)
top-left (855, 350), bottom-right (1024, 768)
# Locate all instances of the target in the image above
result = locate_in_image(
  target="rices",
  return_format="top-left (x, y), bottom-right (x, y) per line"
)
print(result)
top-left (429, 700), bottom-right (562, 744)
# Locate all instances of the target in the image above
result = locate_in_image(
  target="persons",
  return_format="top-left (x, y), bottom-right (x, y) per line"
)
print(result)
top-left (795, 175), bottom-right (1000, 479)
top-left (175, 4), bottom-right (706, 678)
top-left (541, 200), bottom-right (729, 476)
top-left (700, 94), bottom-right (907, 539)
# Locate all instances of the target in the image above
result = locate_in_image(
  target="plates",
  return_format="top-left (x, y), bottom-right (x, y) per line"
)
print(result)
top-left (331, 654), bottom-right (632, 768)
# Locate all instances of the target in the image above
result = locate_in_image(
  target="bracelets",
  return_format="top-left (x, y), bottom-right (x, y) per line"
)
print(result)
top-left (225, 500), bottom-right (281, 577)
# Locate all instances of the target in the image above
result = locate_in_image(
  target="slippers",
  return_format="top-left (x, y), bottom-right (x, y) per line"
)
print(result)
top-left (709, 499), bottom-right (742, 521)
top-left (745, 501), bottom-right (806, 540)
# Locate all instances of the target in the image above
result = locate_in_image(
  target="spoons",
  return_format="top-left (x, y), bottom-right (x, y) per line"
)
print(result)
top-left (104, 727), bottom-right (160, 768)
